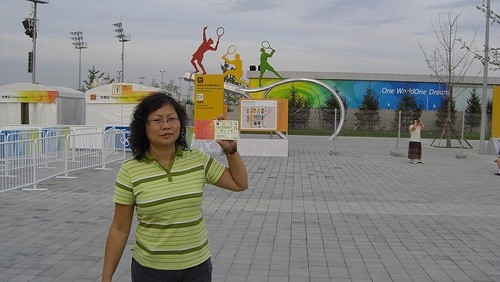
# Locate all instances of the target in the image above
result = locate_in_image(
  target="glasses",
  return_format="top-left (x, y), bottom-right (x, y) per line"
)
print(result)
top-left (146, 116), bottom-right (182, 127)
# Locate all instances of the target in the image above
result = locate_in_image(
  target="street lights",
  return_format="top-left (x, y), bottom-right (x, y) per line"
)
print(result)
top-left (113, 22), bottom-right (131, 83)
top-left (21, 0), bottom-right (49, 84)
top-left (70, 32), bottom-right (88, 91)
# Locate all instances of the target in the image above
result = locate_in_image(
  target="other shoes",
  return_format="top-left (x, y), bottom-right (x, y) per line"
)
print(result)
top-left (418, 161), bottom-right (422, 163)
top-left (410, 161), bottom-right (413, 163)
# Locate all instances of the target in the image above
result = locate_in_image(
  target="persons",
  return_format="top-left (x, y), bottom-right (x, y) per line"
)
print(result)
top-left (102, 92), bottom-right (249, 282)
top-left (408, 118), bottom-right (423, 163)
top-left (494, 152), bottom-right (500, 175)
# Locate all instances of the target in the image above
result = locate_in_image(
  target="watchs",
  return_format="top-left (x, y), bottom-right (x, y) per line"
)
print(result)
top-left (223, 142), bottom-right (237, 155)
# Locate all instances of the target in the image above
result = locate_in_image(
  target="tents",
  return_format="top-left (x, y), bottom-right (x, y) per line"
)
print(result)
top-left (85, 83), bottom-right (177, 152)
top-left (0, 81), bottom-right (85, 150)
